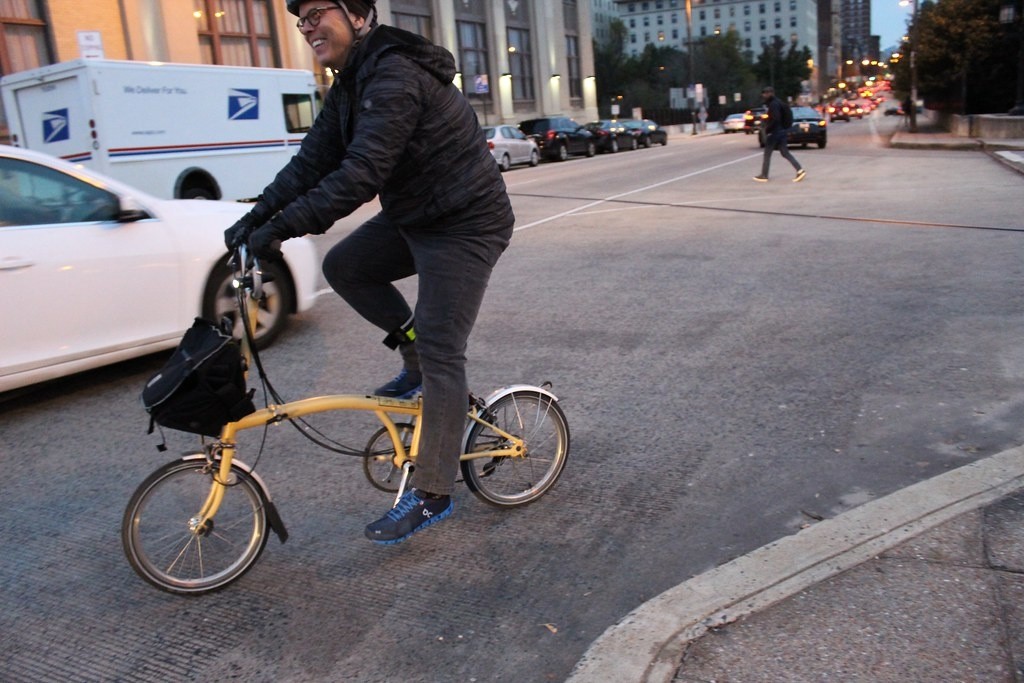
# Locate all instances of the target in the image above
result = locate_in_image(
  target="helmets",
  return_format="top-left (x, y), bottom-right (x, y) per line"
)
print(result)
top-left (287, 0), bottom-right (377, 27)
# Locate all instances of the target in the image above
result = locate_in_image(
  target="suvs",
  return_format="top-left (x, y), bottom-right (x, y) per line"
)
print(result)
top-left (742, 107), bottom-right (769, 134)
top-left (516, 115), bottom-right (600, 164)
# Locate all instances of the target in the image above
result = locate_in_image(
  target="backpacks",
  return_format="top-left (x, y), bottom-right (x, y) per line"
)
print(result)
top-left (776, 99), bottom-right (793, 129)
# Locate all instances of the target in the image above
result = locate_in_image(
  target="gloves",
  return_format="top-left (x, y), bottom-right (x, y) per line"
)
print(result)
top-left (250, 214), bottom-right (290, 260)
top-left (224, 212), bottom-right (258, 253)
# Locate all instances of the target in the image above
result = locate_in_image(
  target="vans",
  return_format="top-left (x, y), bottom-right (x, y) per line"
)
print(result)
top-left (0, 56), bottom-right (333, 214)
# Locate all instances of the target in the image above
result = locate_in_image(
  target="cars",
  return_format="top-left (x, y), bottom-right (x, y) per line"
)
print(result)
top-left (482, 124), bottom-right (542, 172)
top-left (723, 113), bottom-right (745, 134)
top-left (0, 142), bottom-right (323, 404)
top-left (619, 118), bottom-right (668, 148)
top-left (581, 119), bottom-right (639, 154)
top-left (758, 106), bottom-right (828, 149)
top-left (821, 81), bottom-right (923, 123)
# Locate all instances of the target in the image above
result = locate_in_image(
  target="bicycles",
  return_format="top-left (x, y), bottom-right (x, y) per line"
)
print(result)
top-left (121, 239), bottom-right (571, 598)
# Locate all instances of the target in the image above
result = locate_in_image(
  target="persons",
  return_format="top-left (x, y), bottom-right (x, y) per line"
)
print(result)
top-left (753, 88), bottom-right (806, 182)
top-left (223, 0), bottom-right (515, 544)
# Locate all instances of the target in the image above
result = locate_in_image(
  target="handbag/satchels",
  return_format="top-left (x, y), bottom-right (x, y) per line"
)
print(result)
top-left (143, 317), bottom-right (257, 439)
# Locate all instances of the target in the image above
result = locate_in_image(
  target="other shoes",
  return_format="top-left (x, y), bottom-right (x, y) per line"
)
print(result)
top-left (753, 175), bottom-right (768, 182)
top-left (792, 170), bottom-right (806, 182)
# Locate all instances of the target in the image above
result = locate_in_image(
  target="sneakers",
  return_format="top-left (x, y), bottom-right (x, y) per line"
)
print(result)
top-left (364, 487), bottom-right (455, 544)
top-left (371, 367), bottom-right (422, 398)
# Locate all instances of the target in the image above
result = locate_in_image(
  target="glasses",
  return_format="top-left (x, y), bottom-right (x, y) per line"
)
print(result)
top-left (296, 6), bottom-right (341, 27)
